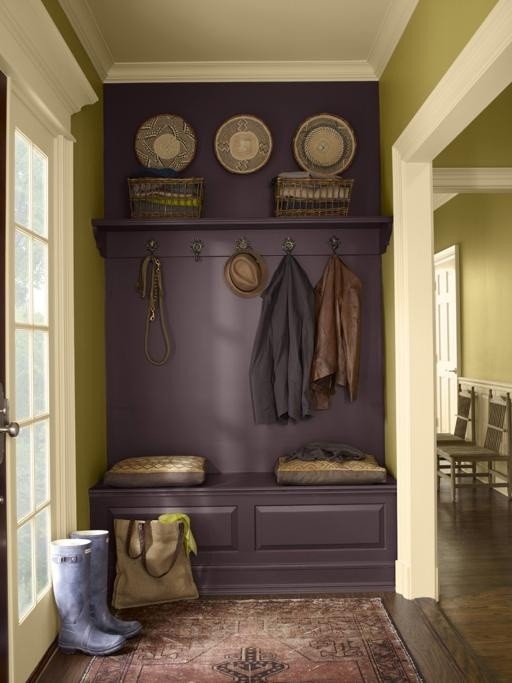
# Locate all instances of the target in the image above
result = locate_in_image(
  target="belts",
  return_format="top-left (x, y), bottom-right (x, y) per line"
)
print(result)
top-left (139, 253), bottom-right (170, 365)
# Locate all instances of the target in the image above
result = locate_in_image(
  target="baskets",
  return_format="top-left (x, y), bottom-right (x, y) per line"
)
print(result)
top-left (125, 175), bottom-right (205, 220)
top-left (272, 176), bottom-right (354, 218)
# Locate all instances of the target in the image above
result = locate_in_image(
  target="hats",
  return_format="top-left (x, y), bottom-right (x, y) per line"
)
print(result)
top-left (224, 249), bottom-right (268, 299)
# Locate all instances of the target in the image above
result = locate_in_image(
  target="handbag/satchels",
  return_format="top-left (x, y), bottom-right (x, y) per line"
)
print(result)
top-left (112, 516), bottom-right (200, 609)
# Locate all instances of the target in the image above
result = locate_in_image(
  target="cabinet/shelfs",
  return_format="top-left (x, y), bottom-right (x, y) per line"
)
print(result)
top-left (89, 81), bottom-right (397, 595)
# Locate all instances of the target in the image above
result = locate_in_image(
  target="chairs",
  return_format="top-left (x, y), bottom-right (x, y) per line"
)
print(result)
top-left (437, 384), bottom-right (476, 479)
top-left (437, 388), bottom-right (512, 503)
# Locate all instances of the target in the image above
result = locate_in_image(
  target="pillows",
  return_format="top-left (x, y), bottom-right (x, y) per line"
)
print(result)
top-left (101, 455), bottom-right (206, 488)
top-left (273, 452), bottom-right (386, 485)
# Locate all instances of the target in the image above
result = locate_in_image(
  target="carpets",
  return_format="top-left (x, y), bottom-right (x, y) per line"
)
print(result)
top-left (79, 598), bottom-right (424, 683)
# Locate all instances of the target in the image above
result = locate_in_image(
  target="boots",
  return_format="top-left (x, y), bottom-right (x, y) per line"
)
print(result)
top-left (70, 529), bottom-right (143, 640)
top-left (47, 538), bottom-right (124, 658)
